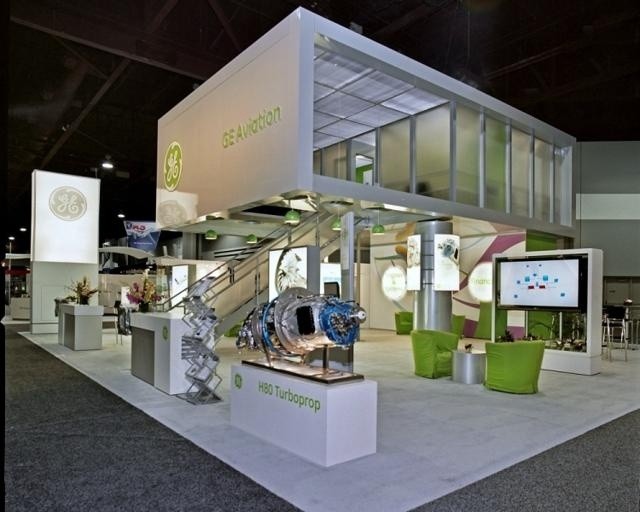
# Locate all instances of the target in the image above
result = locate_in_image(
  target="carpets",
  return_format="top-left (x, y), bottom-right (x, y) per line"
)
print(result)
top-left (17, 329), bottom-right (640, 510)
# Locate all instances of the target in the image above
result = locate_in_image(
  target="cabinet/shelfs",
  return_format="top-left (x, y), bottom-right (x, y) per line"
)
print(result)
top-left (240, 339), bottom-right (363, 382)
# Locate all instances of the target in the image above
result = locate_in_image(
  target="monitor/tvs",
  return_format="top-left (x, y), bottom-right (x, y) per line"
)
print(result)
top-left (496, 253), bottom-right (588, 313)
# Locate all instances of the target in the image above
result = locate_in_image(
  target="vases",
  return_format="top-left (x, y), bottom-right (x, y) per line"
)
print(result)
top-left (139, 302), bottom-right (149, 313)
top-left (80, 296), bottom-right (89, 305)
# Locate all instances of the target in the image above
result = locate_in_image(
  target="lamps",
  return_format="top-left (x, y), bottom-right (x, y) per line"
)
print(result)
top-left (205, 220), bottom-right (217, 240)
top-left (366, 207), bottom-right (391, 235)
top-left (284, 199), bottom-right (300, 225)
top-left (246, 222), bottom-right (257, 244)
top-left (328, 200), bottom-right (353, 231)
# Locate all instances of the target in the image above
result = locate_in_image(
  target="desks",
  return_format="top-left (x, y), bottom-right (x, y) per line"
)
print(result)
top-left (129, 311), bottom-right (216, 396)
top-left (57, 302), bottom-right (104, 350)
top-left (230, 363), bottom-right (378, 467)
top-left (451, 348), bottom-right (487, 385)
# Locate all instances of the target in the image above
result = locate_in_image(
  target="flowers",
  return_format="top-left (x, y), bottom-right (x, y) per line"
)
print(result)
top-left (119, 274), bottom-right (166, 304)
top-left (64, 275), bottom-right (102, 299)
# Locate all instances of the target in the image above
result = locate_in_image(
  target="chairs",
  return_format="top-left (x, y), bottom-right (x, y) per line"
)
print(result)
top-left (452, 313), bottom-right (465, 339)
top-left (411, 329), bottom-right (460, 379)
top-left (601, 304), bottom-right (640, 362)
top-left (394, 311), bottom-right (414, 335)
top-left (482, 339), bottom-right (545, 395)
